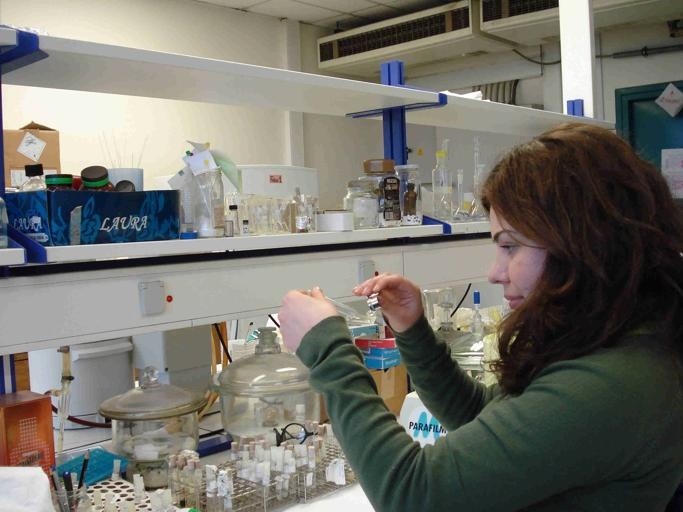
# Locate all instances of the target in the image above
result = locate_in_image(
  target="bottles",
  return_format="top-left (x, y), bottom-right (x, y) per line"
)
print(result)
top-left (50, 419), bottom-right (327, 512)
top-left (17, 162), bottom-right (136, 192)
top-left (470, 289), bottom-right (484, 343)
top-left (190, 150), bottom-right (498, 236)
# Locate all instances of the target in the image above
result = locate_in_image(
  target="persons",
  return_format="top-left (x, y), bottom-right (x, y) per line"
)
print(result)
top-left (274, 122), bottom-right (681, 510)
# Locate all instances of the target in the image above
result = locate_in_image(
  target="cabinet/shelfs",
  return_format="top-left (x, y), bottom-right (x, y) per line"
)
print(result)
top-left (0, 29), bottom-right (614, 271)
